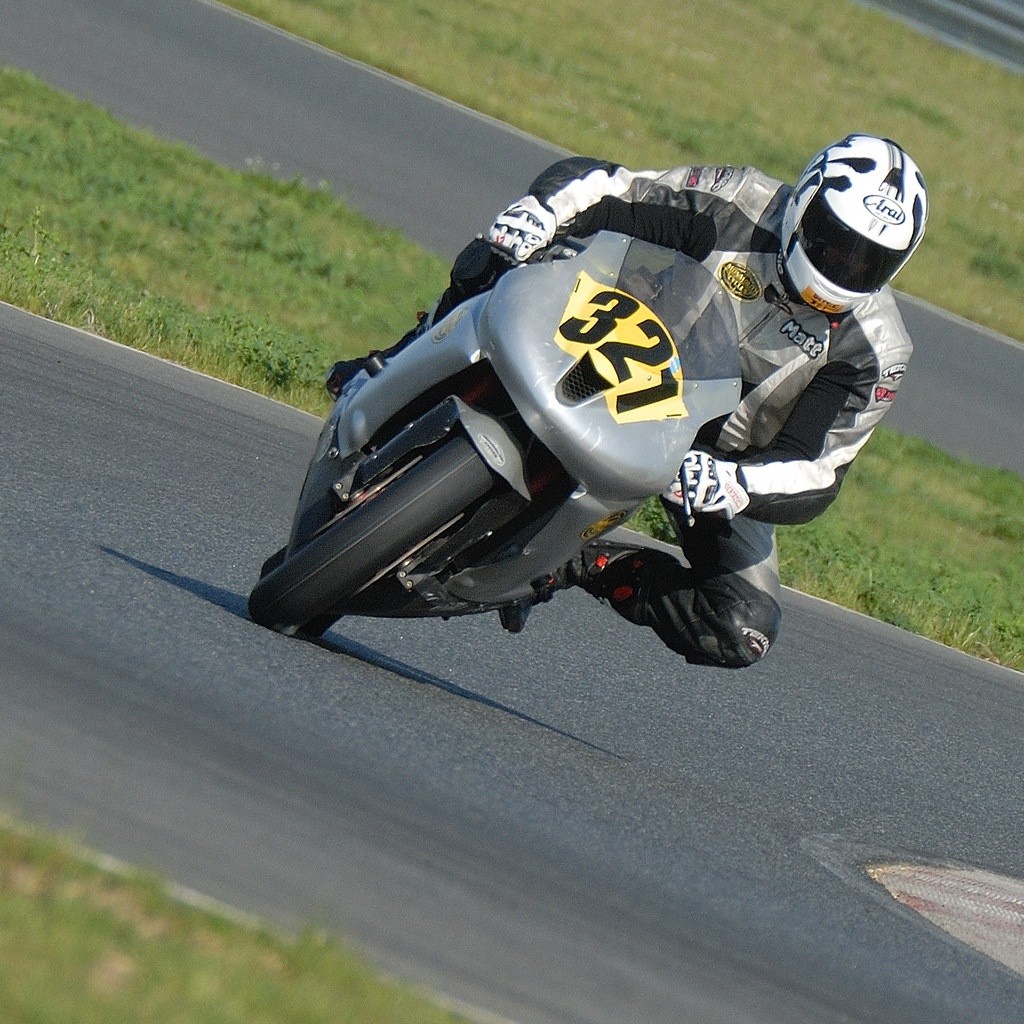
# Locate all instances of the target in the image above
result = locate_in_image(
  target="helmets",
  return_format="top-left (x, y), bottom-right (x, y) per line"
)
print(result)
top-left (780, 134), bottom-right (929, 314)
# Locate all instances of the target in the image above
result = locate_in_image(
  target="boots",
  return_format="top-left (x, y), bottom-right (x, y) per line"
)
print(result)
top-left (499, 538), bottom-right (680, 635)
top-left (325, 286), bottom-right (441, 402)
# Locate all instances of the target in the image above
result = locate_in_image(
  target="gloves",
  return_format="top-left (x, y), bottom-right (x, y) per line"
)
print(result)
top-left (662, 450), bottom-right (750, 521)
top-left (489, 195), bottom-right (558, 266)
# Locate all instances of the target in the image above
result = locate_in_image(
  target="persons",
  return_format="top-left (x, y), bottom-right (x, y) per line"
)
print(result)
top-left (329, 132), bottom-right (929, 669)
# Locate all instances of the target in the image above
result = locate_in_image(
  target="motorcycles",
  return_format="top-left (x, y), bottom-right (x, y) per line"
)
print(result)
top-left (246, 228), bottom-right (754, 639)
